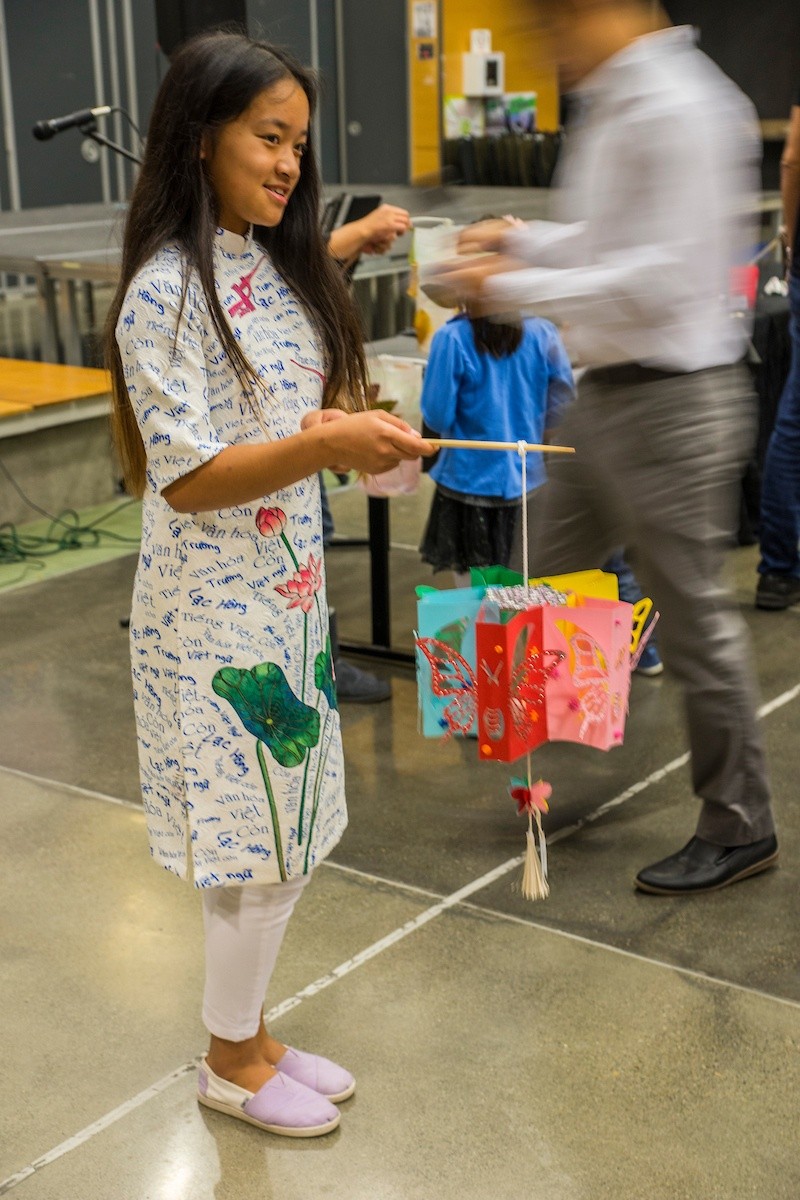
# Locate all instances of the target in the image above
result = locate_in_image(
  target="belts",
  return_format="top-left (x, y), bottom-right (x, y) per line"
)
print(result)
top-left (594, 360), bottom-right (666, 388)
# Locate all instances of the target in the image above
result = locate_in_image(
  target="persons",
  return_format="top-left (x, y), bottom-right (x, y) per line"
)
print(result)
top-left (156, 0), bottom-right (410, 706)
top-left (103, 34), bottom-right (434, 1138)
top-left (419, 0), bottom-right (800, 895)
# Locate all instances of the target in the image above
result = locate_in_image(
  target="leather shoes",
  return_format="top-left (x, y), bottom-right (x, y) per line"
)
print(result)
top-left (634, 832), bottom-right (780, 897)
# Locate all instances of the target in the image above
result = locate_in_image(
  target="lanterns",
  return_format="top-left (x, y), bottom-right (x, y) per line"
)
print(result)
top-left (415, 437), bottom-right (660, 901)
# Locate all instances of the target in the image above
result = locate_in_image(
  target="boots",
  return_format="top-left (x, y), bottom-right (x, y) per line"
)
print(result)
top-left (328, 605), bottom-right (392, 704)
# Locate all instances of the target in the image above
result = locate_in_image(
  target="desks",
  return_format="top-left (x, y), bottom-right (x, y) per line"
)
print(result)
top-left (0, 358), bottom-right (115, 439)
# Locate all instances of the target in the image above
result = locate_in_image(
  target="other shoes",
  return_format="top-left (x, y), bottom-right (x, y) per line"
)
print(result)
top-left (632, 645), bottom-right (663, 675)
top-left (754, 574), bottom-right (800, 613)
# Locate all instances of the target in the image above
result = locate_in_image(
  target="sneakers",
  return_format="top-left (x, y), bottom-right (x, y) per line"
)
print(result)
top-left (194, 1054), bottom-right (341, 1136)
top-left (270, 1043), bottom-right (355, 1102)
